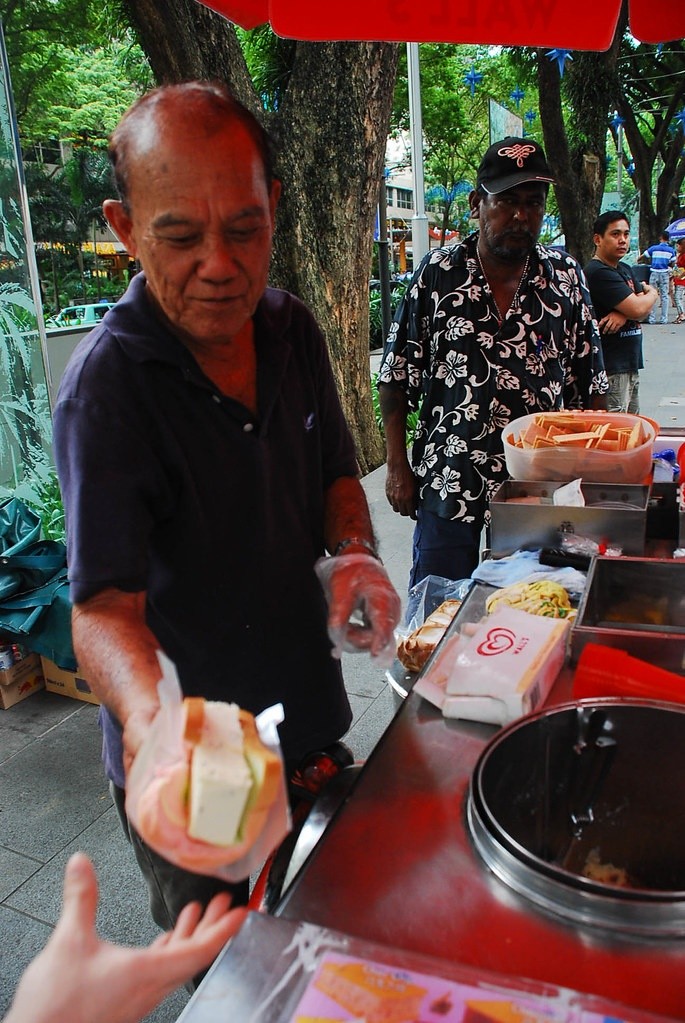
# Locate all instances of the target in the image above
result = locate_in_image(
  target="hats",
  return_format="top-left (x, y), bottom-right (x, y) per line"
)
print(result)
top-left (476, 136), bottom-right (559, 193)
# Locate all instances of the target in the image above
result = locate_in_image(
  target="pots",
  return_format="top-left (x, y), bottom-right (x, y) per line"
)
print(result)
top-left (477, 703), bottom-right (685, 899)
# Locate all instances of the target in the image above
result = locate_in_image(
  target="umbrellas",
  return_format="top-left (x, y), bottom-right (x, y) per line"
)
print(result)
top-left (665, 218), bottom-right (685, 239)
top-left (196, 0), bottom-right (685, 50)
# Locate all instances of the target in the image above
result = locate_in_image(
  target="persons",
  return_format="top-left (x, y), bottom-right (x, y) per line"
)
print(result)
top-left (376, 138), bottom-right (610, 629)
top-left (52, 82), bottom-right (401, 995)
top-left (637, 231), bottom-right (685, 324)
top-left (3, 853), bottom-right (243, 1023)
top-left (584, 211), bottom-right (658, 415)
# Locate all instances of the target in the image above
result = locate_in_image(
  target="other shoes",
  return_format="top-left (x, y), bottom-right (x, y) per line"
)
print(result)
top-left (672, 313), bottom-right (685, 324)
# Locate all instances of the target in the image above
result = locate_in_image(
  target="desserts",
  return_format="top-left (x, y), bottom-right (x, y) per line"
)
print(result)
top-left (293, 960), bottom-right (561, 1023)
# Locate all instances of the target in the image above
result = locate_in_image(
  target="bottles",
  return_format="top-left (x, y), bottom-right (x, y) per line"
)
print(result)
top-left (662, 449), bottom-right (680, 481)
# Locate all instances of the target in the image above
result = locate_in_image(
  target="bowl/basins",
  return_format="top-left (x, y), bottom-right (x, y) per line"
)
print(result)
top-left (499, 411), bottom-right (656, 484)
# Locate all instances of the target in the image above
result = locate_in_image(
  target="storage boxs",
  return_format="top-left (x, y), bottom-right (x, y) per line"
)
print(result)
top-left (0, 652), bottom-right (101, 711)
top-left (411, 475), bottom-right (685, 729)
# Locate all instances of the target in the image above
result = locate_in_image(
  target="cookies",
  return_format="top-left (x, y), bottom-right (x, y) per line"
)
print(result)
top-left (506, 412), bottom-right (655, 484)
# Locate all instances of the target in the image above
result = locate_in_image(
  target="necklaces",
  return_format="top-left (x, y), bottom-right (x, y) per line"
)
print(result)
top-left (477, 246), bottom-right (530, 321)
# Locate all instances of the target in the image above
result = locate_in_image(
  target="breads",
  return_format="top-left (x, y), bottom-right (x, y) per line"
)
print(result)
top-left (174, 695), bottom-right (282, 849)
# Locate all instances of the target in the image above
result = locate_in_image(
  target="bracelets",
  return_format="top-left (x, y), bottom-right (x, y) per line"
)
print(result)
top-left (334, 538), bottom-right (382, 564)
